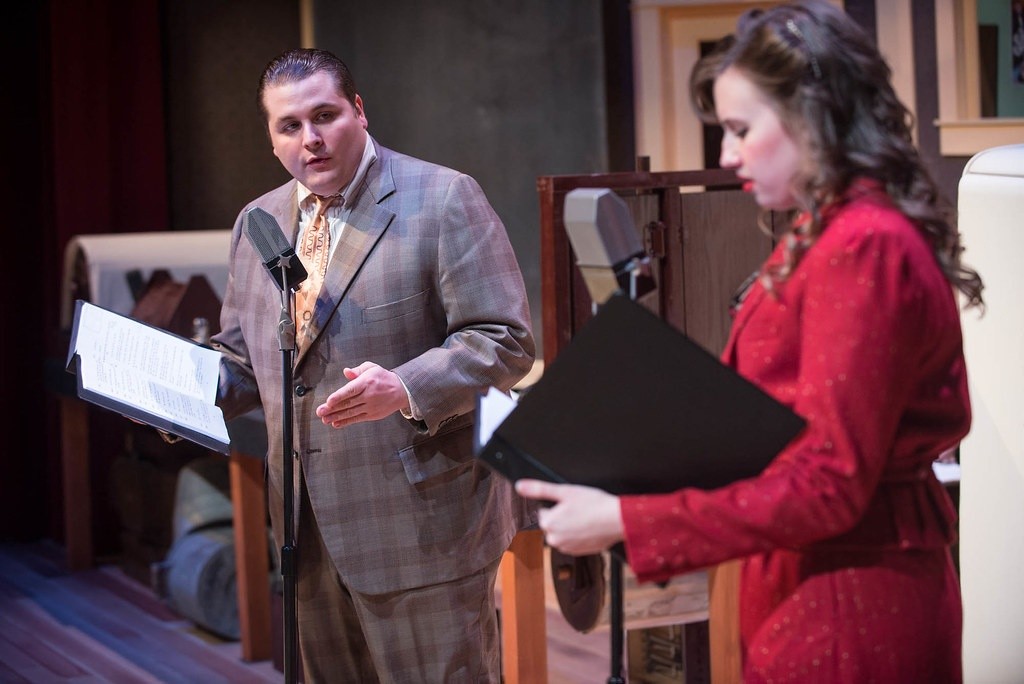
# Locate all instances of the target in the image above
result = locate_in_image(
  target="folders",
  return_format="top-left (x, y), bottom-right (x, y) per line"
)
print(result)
top-left (470, 295), bottom-right (806, 585)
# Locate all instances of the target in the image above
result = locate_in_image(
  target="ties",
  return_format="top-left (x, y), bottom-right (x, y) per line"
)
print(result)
top-left (289, 195), bottom-right (340, 349)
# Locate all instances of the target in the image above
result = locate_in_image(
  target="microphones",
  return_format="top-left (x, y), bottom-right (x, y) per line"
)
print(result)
top-left (242, 206), bottom-right (308, 351)
top-left (562, 187), bottom-right (661, 315)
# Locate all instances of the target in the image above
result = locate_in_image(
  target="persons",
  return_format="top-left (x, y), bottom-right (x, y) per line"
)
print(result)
top-left (514, 2), bottom-right (984, 684)
top-left (126, 48), bottom-right (536, 684)
